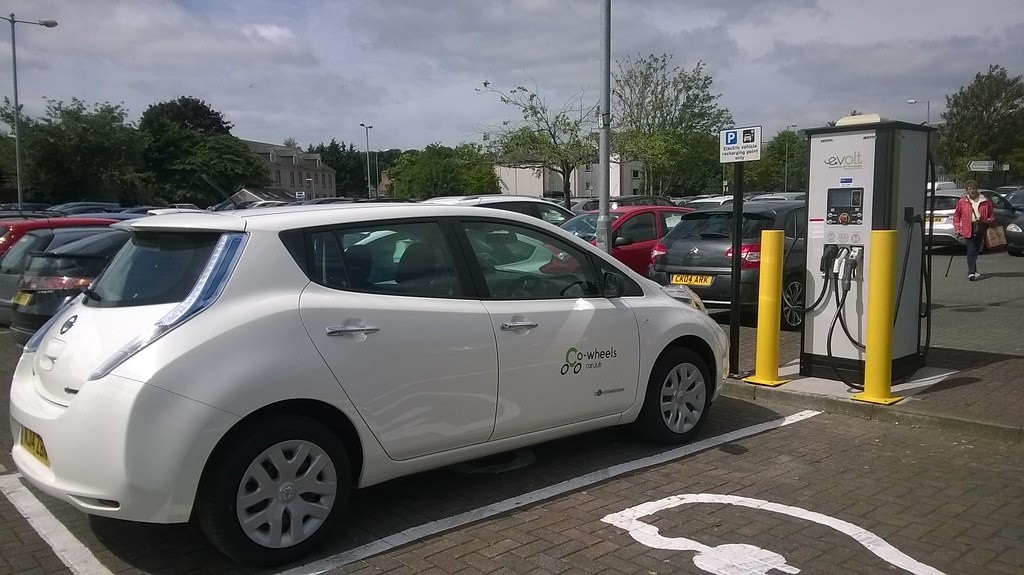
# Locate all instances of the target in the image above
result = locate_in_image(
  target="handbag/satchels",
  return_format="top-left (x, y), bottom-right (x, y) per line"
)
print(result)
top-left (984, 222), bottom-right (1008, 250)
top-left (972, 219), bottom-right (986, 235)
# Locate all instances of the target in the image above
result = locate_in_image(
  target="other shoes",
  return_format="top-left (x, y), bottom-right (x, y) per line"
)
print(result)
top-left (974, 272), bottom-right (980, 277)
top-left (968, 273), bottom-right (975, 281)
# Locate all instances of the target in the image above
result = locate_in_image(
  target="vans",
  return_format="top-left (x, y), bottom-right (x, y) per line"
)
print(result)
top-left (927, 180), bottom-right (957, 193)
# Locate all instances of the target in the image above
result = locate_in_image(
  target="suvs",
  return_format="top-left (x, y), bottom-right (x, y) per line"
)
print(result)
top-left (7, 230), bottom-right (133, 351)
top-left (647, 200), bottom-right (810, 331)
top-left (926, 188), bottom-right (1024, 255)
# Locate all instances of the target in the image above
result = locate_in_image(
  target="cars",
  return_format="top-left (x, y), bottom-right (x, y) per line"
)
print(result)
top-left (0, 192), bottom-right (806, 285)
top-left (1005, 214), bottom-right (1024, 258)
top-left (541, 207), bottom-right (697, 280)
top-left (992, 185), bottom-right (1024, 204)
top-left (7, 204), bottom-right (730, 572)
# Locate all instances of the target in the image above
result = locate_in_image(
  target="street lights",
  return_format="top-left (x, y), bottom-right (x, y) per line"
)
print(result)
top-left (359, 122), bottom-right (374, 198)
top-left (906, 98), bottom-right (930, 127)
top-left (0, 13), bottom-right (60, 213)
top-left (783, 123), bottom-right (798, 192)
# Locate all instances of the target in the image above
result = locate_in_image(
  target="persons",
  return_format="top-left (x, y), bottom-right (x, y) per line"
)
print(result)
top-left (953, 180), bottom-right (995, 280)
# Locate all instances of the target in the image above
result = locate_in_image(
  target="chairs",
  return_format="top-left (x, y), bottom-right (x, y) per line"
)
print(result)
top-left (394, 243), bottom-right (437, 297)
top-left (345, 244), bottom-right (381, 290)
top-left (623, 223), bottom-right (651, 239)
